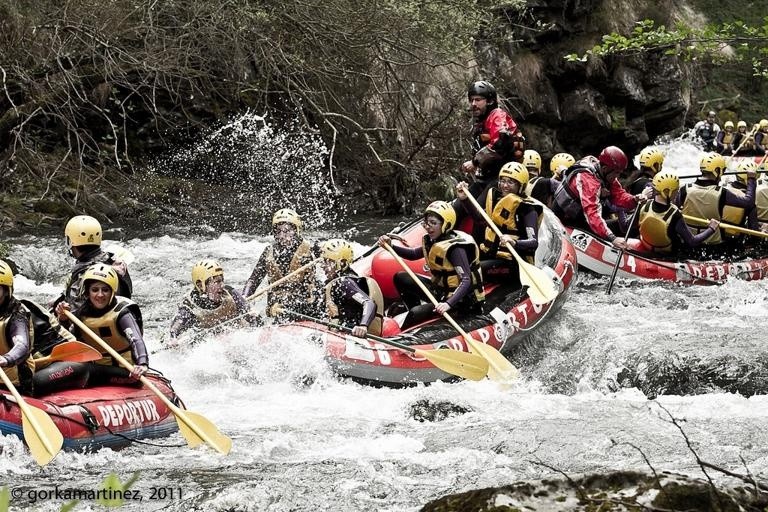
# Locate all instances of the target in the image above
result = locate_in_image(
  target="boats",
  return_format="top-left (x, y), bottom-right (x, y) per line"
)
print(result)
top-left (703, 152), bottom-right (768, 180)
top-left (236, 193), bottom-right (572, 385)
top-left (0, 369), bottom-right (198, 446)
top-left (544, 198), bottom-right (767, 284)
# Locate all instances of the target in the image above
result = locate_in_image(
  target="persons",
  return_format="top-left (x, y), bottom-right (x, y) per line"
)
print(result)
top-left (692, 111), bottom-right (768, 154)
top-left (279, 240), bottom-right (384, 342)
top-left (48, 214), bottom-right (133, 315)
top-left (56, 264), bottom-right (148, 387)
top-left (242, 209), bottom-right (318, 322)
top-left (452, 160), bottom-right (543, 288)
top-left (379, 200), bottom-right (486, 331)
top-left (526, 151), bottom-right (767, 261)
top-left (14, 295), bottom-right (91, 396)
top-left (0, 259), bottom-right (34, 407)
top-left (164, 259), bottom-right (264, 348)
top-left (450, 81), bottom-right (526, 242)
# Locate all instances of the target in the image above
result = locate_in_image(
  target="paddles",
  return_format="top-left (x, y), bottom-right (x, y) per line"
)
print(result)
top-left (270, 303), bottom-right (489, 381)
top-left (0, 368), bottom-right (64, 467)
top-left (382, 241), bottom-right (523, 381)
top-left (462, 186), bottom-right (557, 305)
top-left (62, 308), bottom-right (230, 455)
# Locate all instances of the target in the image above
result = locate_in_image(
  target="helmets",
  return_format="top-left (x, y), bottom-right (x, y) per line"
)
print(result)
top-left (499, 146), bottom-right (768, 200)
top-left (65, 215), bottom-right (102, 251)
top-left (468, 81), bottom-right (496, 98)
top-left (321, 239), bottom-right (352, 271)
top-left (80, 264), bottom-right (119, 306)
top-left (425, 200), bottom-right (457, 234)
top-left (272, 209), bottom-right (301, 237)
top-left (0, 260), bottom-right (14, 299)
top-left (706, 111), bottom-right (768, 131)
top-left (192, 260), bottom-right (225, 293)
